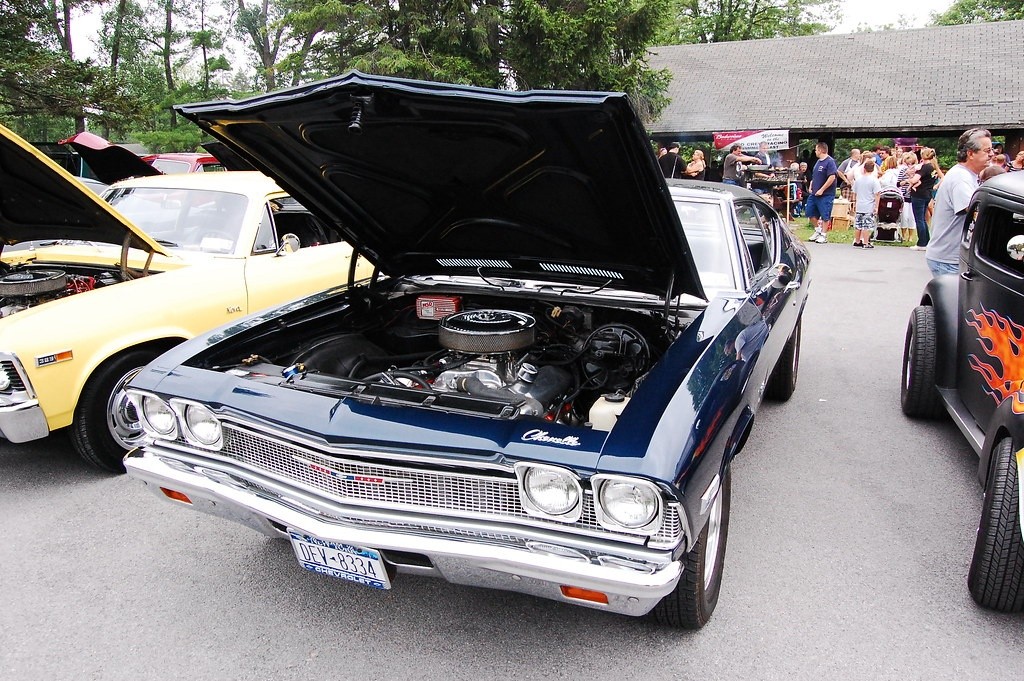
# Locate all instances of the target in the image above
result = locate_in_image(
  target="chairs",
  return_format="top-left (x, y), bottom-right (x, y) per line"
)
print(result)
top-left (273, 211), bottom-right (329, 245)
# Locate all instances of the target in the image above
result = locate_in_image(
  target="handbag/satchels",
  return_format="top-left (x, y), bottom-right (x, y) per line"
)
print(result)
top-left (840, 182), bottom-right (851, 196)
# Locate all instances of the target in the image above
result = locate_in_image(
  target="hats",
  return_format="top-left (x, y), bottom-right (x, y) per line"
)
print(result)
top-left (670, 142), bottom-right (679, 148)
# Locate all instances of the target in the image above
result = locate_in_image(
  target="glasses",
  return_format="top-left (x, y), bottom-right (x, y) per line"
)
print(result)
top-left (961, 129), bottom-right (978, 148)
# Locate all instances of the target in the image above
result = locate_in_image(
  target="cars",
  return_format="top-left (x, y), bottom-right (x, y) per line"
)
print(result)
top-left (0, 124), bottom-right (381, 472)
top-left (899, 166), bottom-right (1023, 620)
top-left (29, 138), bottom-right (225, 196)
top-left (105, 73), bottom-right (810, 631)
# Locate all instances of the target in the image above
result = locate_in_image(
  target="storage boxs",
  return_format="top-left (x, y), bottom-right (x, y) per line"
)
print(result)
top-left (818, 199), bottom-right (851, 232)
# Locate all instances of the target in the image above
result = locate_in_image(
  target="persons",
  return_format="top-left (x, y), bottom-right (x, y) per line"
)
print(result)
top-left (837, 145), bottom-right (948, 251)
top-left (703, 144), bottom-right (762, 186)
top-left (989, 143), bottom-right (1024, 174)
top-left (753, 141), bottom-right (776, 194)
top-left (924, 127), bottom-right (994, 280)
top-left (851, 160), bottom-right (881, 249)
top-left (1016, 247), bottom-right (1021, 260)
top-left (685, 149), bottom-right (706, 180)
top-left (971, 166), bottom-right (1007, 184)
top-left (805, 142), bottom-right (838, 244)
top-left (790, 149), bottom-right (821, 218)
top-left (658, 142), bottom-right (686, 179)
top-left (724, 321), bottom-right (771, 362)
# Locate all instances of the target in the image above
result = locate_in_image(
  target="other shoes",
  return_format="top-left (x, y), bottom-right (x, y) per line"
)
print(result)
top-left (909, 245), bottom-right (926, 251)
top-left (853, 240), bottom-right (863, 246)
top-left (862, 243), bottom-right (874, 248)
top-left (809, 232), bottom-right (821, 241)
top-left (816, 235), bottom-right (828, 243)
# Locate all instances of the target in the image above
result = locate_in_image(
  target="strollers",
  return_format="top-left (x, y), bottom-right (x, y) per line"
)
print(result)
top-left (869, 189), bottom-right (905, 243)
